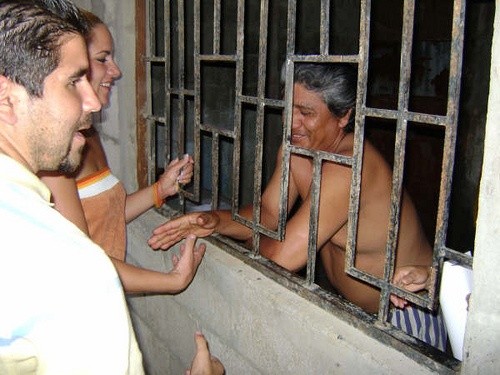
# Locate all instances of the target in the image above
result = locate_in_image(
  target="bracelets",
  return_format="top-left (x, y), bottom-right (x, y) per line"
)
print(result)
top-left (153, 176), bottom-right (163, 209)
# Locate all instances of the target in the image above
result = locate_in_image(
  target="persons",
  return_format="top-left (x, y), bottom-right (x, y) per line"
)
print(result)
top-left (1, 0), bottom-right (229, 375)
top-left (390, 260), bottom-right (433, 311)
top-left (150, 57), bottom-right (447, 356)
top-left (42, 1), bottom-right (204, 296)
